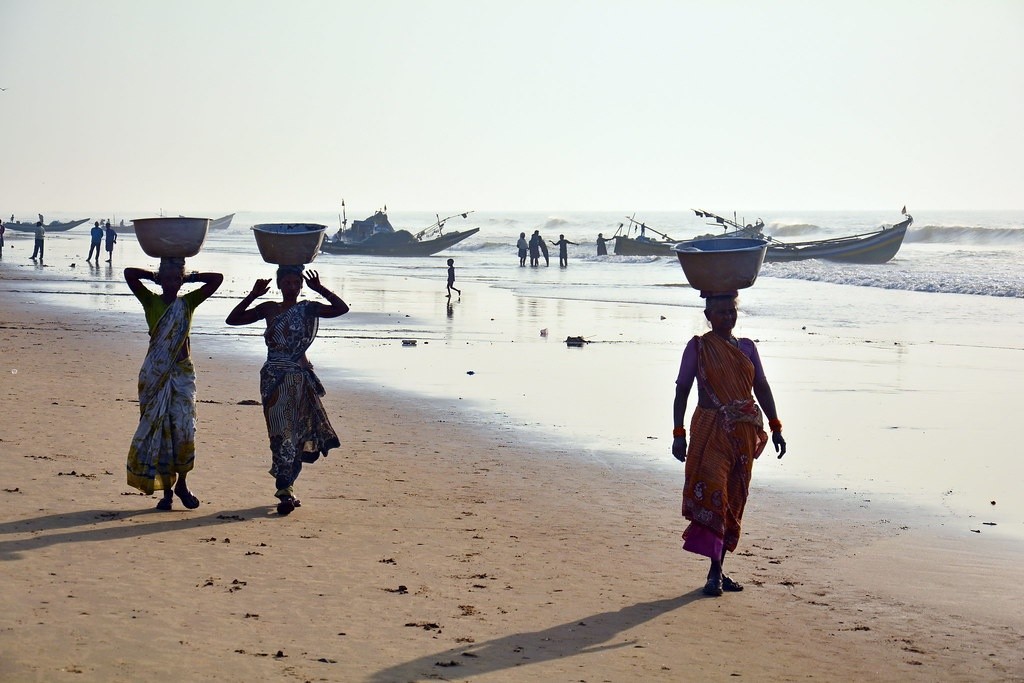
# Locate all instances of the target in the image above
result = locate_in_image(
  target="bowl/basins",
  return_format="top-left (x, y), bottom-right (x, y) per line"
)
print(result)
top-left (249, 222), bottom-right (327, 265)
top-left (131, 218), bottom-right (212, 257)
top-left (669, 237), bottom-right (769, 290)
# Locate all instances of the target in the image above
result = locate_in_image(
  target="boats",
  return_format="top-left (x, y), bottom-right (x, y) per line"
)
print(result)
top-left (2, 214), bottom-right (91, 232)
top-left (613, 206), bottom-right (914, 264)
top-left (100, 212), bottom-right (236, 234)
top-left (319, 198), bottom-right (481, 255)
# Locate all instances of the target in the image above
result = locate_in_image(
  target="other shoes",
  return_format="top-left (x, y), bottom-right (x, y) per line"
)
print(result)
top-left (156, 498), bottom-right (173, 510)
top-left (174, 488), bottom-right (199, 509)
top-left (721, 576), bottom-right (744, 591)
top-left (277, 497), bottom-right (294, 516)
top-left (290, 495), bottom-right (300, 507)
top-left (704, 573), bottom-right (724, 595)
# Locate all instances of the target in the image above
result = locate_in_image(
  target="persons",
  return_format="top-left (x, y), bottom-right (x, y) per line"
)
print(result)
top-left (225, 264), bottom-right (349, 515)
top-left (28, 222), bottom-right (45, 259)
top-left (86, 222), bottom-right (103, 262)
top-left (104, 223), bottom-right (118, 262)
top-left (671, 294), bottom-right (787, 596)
top-left (332, 231), bottom-right (348, 248)
top-left (517, 230), bottom-right (550, 268)
top-left (596, 233), bottom-right (613, 256)
top-left (548, 234), bottom-right (580, 268)
top-left (445, 259), bottom-right (461, 298)
top-left (0, 219), bottom-right (5, 260)
top-left (123, 257), bottom-right (224, 512)
